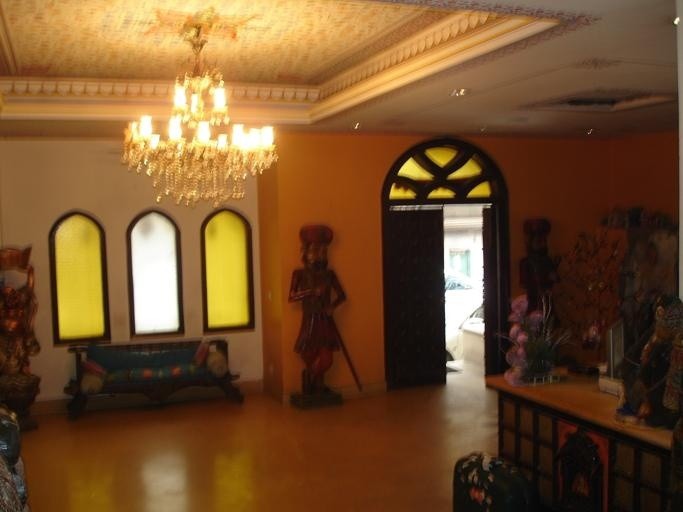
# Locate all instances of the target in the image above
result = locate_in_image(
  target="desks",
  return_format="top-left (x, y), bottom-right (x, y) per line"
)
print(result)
top-left (487, 373), bottom-right (683, 512)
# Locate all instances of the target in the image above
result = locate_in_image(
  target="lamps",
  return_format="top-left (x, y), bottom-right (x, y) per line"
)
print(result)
top-left (120, 7), bottom-right (280, 209)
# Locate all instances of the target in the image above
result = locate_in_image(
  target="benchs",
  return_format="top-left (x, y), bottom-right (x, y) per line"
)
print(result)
top-left (64, 340), bottom-right (244, 421)
